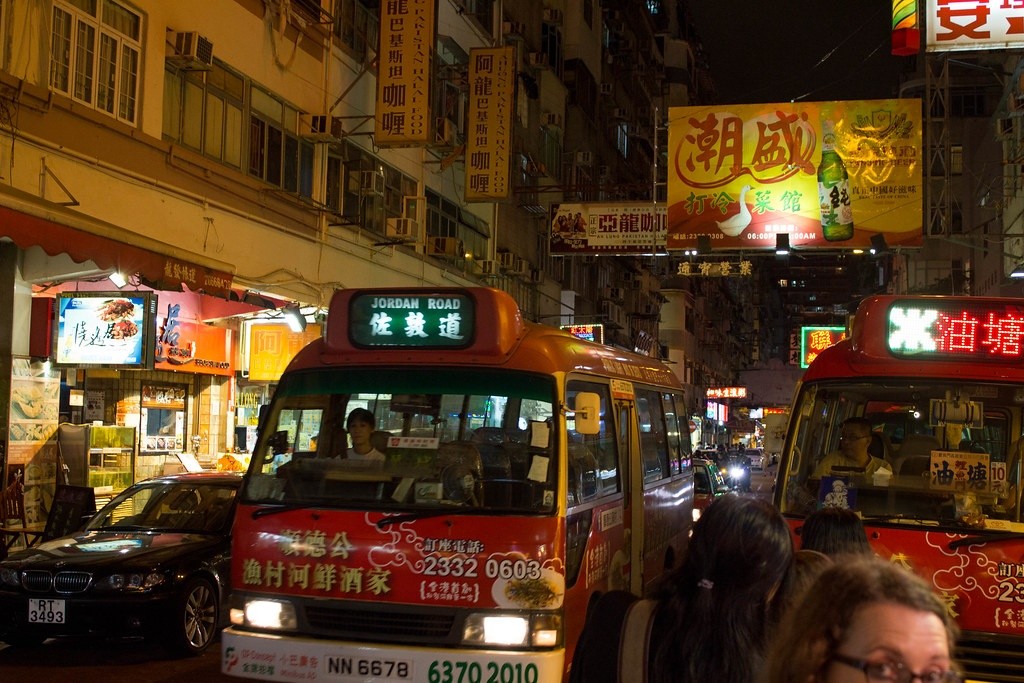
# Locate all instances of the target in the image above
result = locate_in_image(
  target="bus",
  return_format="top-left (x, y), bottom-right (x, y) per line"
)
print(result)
top-left (772, 294), bottom-right (1024, 683)
top-left (221, 284), bottom-right (690, 683)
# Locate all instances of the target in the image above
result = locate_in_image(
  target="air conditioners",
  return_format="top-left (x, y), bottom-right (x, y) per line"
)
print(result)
top-left (166, 31), bottom-right (213, 71)
top-left (662, 346), bottom-right (670, 358)
top-left (601, 82), bottom-right (612, 94)
top-left (602, 301), bottom-right (624, 330)
top-left (435, 117), bottom-right (457, 146)
top-left (550, 8), bottom-right (564, 26)
top-left (428, 237), bottom-right (464, 260)
top-left (300, 114), bottom-right (342, 144)
top-left (386, 218), bottom-right (419, 241)
top-left (599, 166), bottom-right (610, 178)
top-left (503, 19), bottom-right (526, 36)
top-left (578, 152), bottom-right (592, 164)
top-left (349, 170), bottom-right (384, 196)
top-left (533, 269), bottom-right (544, 282)
top-left (530, 52), bottom-right (549, 71)
top-left (622, 273), bottom-right (633, 283)
top-left (616, 107), bottom-right (627, 119)
top-left (515, 260), bottom-right (532, 282)
top-left (632, 280), bottom-right (643, 291)
top-left (501, 252), bottom-right (516, 270)
top-left (544, 113), bottom-right (562, 127)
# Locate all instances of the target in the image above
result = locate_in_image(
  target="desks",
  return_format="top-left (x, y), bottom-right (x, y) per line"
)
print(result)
top-left (0, 521), bottom-right (47, 556)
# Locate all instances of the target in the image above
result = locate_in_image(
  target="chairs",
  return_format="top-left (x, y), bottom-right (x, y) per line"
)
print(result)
top-left (159, 492), bottom-right (232, 530)
top-left (872, 427), bottom-right (1023, 483)
top-left (0, 490), bottom-right (31, 561)
top-left (370, 425), bottom-right (663, 481)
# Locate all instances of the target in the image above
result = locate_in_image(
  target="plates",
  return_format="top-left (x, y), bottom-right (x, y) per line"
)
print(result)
top-left (608, 550), bottom-right (632, 592)
top-left (492, 566), bottom-right (565, 610)
top-left (94, 307), bottom-right (140, 326)
top-left (102, 330), bottom-right (140, 346)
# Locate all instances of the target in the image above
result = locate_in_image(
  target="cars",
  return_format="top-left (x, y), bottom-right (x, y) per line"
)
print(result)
top-left (1, 471), bottom-right (242, 654)
top-left (693, 447), bottom-right (773, 524)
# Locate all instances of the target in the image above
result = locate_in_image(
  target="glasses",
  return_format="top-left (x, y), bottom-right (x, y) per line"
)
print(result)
top-left (824, 651), bottom-right (962, 683)
top-left (839, 432), bottom-right (870, 441)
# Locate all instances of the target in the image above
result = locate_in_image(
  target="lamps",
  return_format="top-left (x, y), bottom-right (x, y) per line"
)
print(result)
top-left (108, 270), bottom-right (130, 290)
top-left (241, 294), bottom-right (275, 310)
top-left (284, 310), bottom-right (308, 333)
top-left (775, 233), bottom-right (790, 254)
top-left (200, 288), bottom-right (239, 303)
top-left (138, 274), bottom-right (184, 293)
top-left (869, 232), bottom-right (889, 253)
top-left (697, 236), bottom-right (712, 254)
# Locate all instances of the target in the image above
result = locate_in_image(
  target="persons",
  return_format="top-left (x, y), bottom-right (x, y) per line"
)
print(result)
top-left (812, 417), bottom-right (892, 486)
top-left (338, 402), bottom-right (387, 475)
top-left (756, 554), bottom-right (966, 683)
top-left (566, 492), bottom-right (833, 683)
top-left (800, 507), bottom-right (870, 554)
top-left (692, 437), bottom-right (746, 469)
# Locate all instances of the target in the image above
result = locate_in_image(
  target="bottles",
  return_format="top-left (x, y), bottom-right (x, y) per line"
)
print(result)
top-left (818, 132), bottom-right (855, 242)
